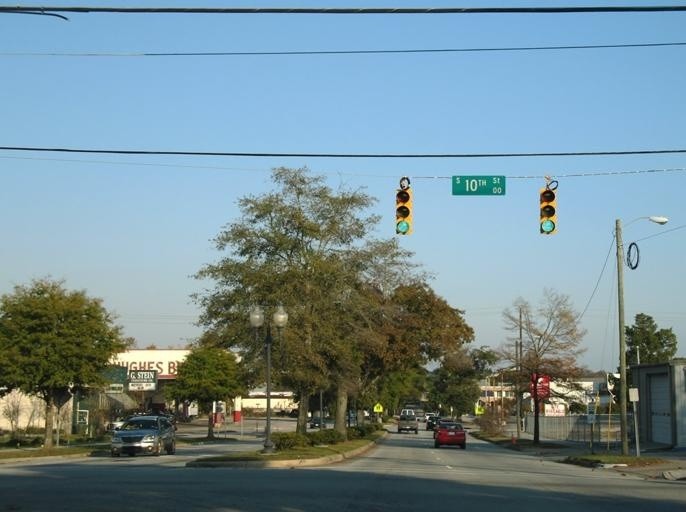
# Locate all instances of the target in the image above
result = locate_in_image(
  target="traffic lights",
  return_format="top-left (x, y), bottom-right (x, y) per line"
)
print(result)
top-left (394, 186), bottom-right (414, 237)
top-left (539, 186), bottom-right (558, 235)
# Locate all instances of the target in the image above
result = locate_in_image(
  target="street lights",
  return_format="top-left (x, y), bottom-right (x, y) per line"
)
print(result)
top-left (251, 303), bottom-right (289, 455)
top-left (613, 211), bottom-right (669, 450)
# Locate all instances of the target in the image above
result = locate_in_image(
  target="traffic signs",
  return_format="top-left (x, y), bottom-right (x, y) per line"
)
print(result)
top-left (452, 176), bottom-right (506, 195)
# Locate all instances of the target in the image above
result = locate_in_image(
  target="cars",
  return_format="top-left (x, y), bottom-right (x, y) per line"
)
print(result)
top-left (107, 407), bottom-right (178, 457)
top-left (397, 406), bottom-right (468, 451)
